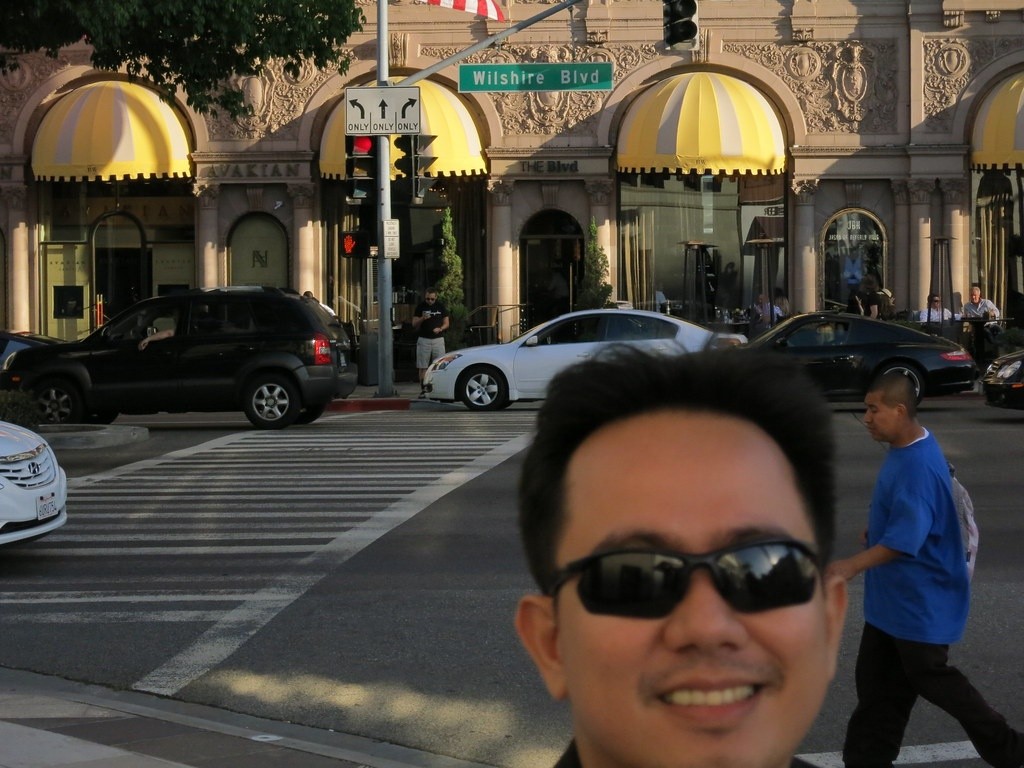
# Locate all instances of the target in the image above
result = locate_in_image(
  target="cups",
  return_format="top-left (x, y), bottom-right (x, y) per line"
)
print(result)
top-left (954, 314), bottom-right (962, 320)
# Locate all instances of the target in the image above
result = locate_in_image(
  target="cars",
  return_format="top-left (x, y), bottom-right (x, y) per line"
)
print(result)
top-left (749, 312), bottom-right (980, 409)
top-left (418, 307), bottom-right (750, 412)
top-left (0, 330), bottom-right (68, 380)
top-left (982, 349), bottom-right (1024, 411)
top-left (0, 421), bottom-right (71, 548)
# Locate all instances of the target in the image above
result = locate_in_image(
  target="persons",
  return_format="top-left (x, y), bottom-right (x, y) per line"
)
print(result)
top-left (861, 274), bottom-right (882, 318)
top-left (828, 374), bottom-right (1024, 768)
top-left (765, 296), bottom-right (790, 325)
top-left (303, 291), bottom-right (313, 300)
top-left (656, 281), bottom-right (668, 312)
top-left (517, 334), bottom-right (847, 768)
top-left (919, 295), bottom-right (953, 322)
top-left (963, 286), bottom-right (1006, 345)
top-left (138, 328), bottom-right (174, 351)
top-left (412, 287), bottom-right (451, 398)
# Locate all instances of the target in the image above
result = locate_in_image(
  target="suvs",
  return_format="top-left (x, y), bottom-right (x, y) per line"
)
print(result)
top-left (0, 285), bottom-right (363, 429)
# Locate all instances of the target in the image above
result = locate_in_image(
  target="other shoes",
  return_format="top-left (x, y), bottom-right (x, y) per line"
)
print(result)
top-left (418, 393), bottom-right (425, 399)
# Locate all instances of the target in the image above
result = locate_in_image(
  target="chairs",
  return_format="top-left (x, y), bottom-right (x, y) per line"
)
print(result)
top-left (834, 323), bottom-right (846, 345)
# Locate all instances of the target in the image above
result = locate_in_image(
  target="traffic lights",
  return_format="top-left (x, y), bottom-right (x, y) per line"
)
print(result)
top-left (344, 130), bottom-right (378, 206)
top-left (661, 0), bottom-right (704, 54)
top-left (339, 232), bottom-right (379, 258)
top-left (407, 130), bottom-right (438, 207)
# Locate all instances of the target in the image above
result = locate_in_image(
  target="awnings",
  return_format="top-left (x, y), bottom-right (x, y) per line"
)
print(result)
top-left (617, 72), bottom-right (787, 175)
top-left (30, 82), bottom-right (192, 183)
top-left (319, 75), bottom-right (487, 179)
top-left (973, 72), bottom-right (1024, 172)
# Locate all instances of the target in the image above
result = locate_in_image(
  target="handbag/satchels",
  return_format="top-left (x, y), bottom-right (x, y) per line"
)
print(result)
top-left (948, 463), bottom-right (979, 582)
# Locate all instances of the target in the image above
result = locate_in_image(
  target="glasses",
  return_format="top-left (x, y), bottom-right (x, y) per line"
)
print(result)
top-left (934, 300), bottom-right (940, 302)
top-left (551, 539), bottom-right (827, 618)
top-left (426, 297), bottom-right (436, 301)
